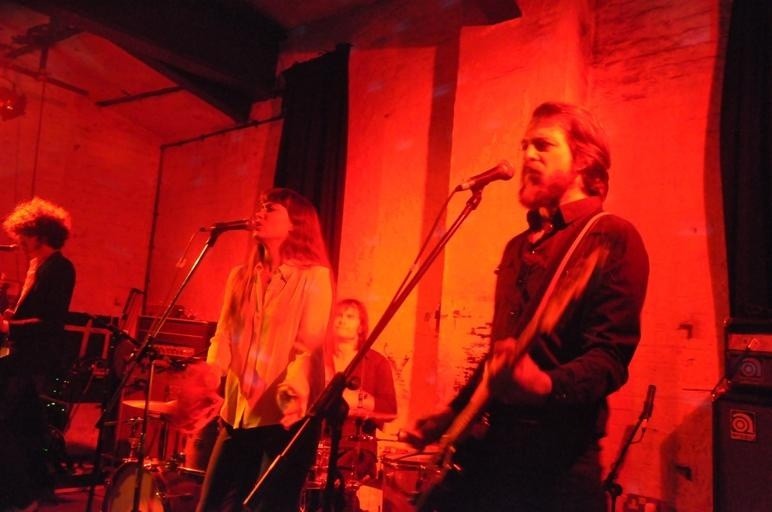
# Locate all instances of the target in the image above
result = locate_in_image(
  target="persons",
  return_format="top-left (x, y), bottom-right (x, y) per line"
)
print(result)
top-left (1, 195), bottom-right (77, 511)
top-left (177, 187), bottom-right (335, 512)
top-left (308, 299), bottom-right (399, 511)
top-left (409, 98), bottom-right (651, 511)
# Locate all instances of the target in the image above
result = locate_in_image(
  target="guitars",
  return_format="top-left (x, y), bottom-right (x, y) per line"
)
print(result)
top-left (415, 246), bottom-right (611, 496)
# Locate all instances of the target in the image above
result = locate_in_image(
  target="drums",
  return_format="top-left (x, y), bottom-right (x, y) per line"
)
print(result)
top-left (382, 453), bottom-right (449, 512)
top-left (102, 461), bottom-right (206, 511)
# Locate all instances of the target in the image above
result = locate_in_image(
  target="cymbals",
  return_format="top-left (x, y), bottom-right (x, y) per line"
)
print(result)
top-left (124, 401), bottom-right (180, 414)
top-left (349, 412), bottom-right (395, 423)
